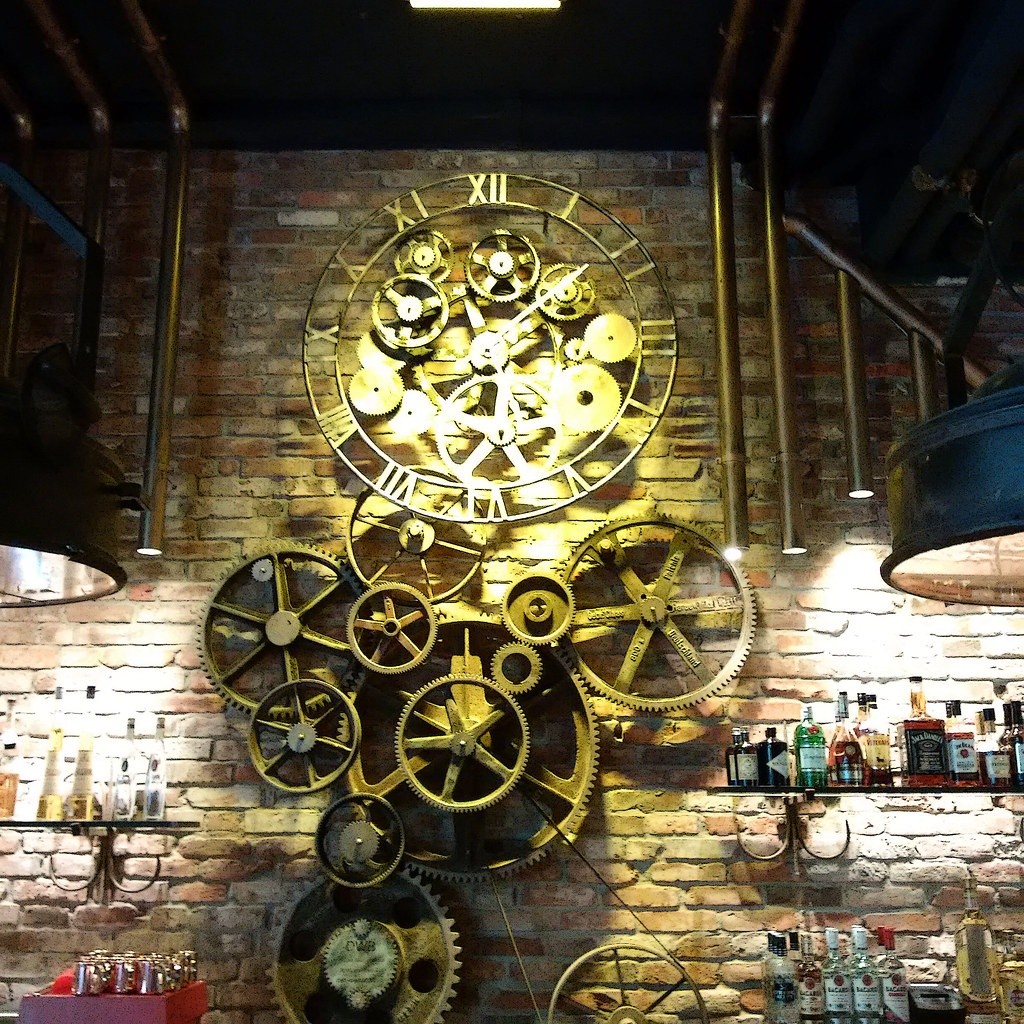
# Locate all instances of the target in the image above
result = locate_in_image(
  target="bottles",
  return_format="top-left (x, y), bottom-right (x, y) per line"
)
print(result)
top-left (107, 717), bottom-right (140, 821)
top-left (142, 712), bottom-right (168, 821)
top-left (1, 698), bottom-right (19, 819)
top-left (717, 666), bottom-right (1024, 793)
top-left (68, 685), bottom-right (96, 820)
top-left (760, 877), bottom-right (1010, 1024)
top-left (36, 685), bottom-right (64, 820)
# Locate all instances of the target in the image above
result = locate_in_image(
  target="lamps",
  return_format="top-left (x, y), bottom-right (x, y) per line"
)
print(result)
top-left (1, 0), bottom-right (192, 614)
top-left (705, 0), bottom-right (1024, 608)
